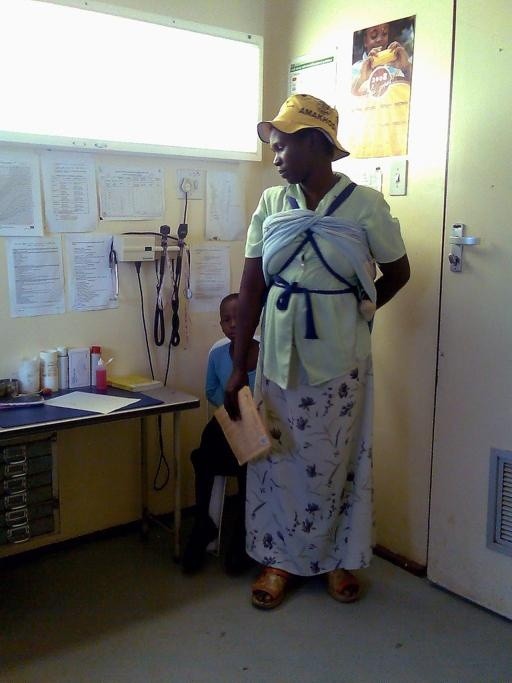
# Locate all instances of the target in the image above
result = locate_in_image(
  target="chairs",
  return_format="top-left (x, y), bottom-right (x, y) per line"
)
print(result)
top-left (208, 338), bottom-right (258, 555)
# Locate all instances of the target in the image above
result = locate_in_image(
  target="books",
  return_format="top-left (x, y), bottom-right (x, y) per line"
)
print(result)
top-left (215, 386), bottom-right (272, 465)
top-left (107, 375), bottom-right (164, 393)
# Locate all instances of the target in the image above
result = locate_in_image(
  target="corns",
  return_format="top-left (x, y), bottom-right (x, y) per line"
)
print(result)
top-left (370, 49), bottom-right (395, 69)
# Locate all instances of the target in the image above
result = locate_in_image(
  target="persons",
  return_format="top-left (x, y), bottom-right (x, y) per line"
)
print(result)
top-left (177, 293), bottom-right (260, 577)
top-left (349, 22), bottom-right (412, 95)
top-left (223, 94), bottom-right (411, 609)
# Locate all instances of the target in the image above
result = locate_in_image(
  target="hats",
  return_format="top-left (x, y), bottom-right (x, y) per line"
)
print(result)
top-left (255, 93), bottom-right (350, 163)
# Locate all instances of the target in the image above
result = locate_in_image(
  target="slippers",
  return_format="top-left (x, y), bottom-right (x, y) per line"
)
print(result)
top-left (247, 560), bottom-right (292, 609)
top-left (323, 562), bottom-right (364, 605)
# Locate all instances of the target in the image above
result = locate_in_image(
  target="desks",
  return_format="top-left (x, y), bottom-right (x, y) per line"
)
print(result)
top-left (0, 378), bottom-right (201, 563)
